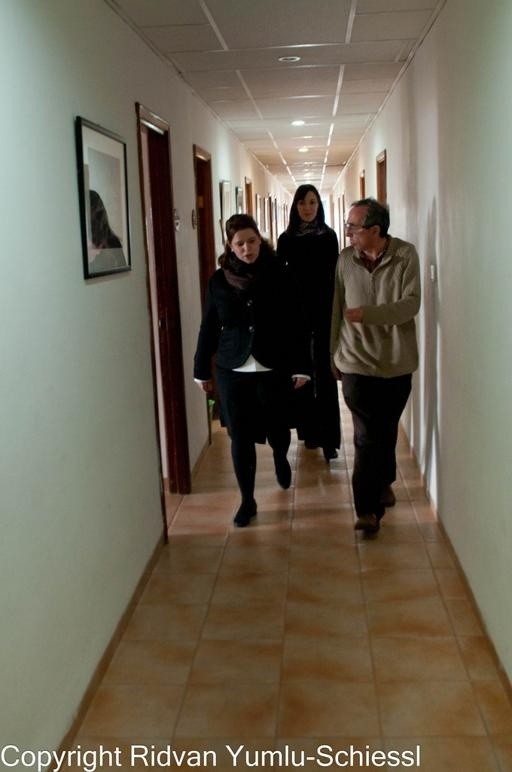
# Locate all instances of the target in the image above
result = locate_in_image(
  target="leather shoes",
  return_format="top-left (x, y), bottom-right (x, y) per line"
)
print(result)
top-left (322, 446), bottom-right (338, 459)
top-left (234, 496), bottom-right (256, 527)
top-left (274, 455), bottom-right (290, 490)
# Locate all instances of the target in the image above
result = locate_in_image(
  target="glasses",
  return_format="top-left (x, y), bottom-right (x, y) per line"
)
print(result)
top-left (344, 223), bottom-right (368, 229)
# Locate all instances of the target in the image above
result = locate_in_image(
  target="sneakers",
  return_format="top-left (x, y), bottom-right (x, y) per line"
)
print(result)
top-left (380, 485), bottom-right (396, 507)
top-left (354, 514), bottom-right (379, 533)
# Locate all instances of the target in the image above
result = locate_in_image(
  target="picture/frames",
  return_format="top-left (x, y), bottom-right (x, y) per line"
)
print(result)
top-left (77, 116), bottom-right (132, 280)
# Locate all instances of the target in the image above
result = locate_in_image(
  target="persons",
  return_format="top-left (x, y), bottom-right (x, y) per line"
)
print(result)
top-left (87, 188), bottom-right (128, 271)
top-left (329, 197), bottom-right (424, 533)
top-left (275, 184), bottom-right (343, 460)
top-left (192, 213), bottom-right (313, 527)
